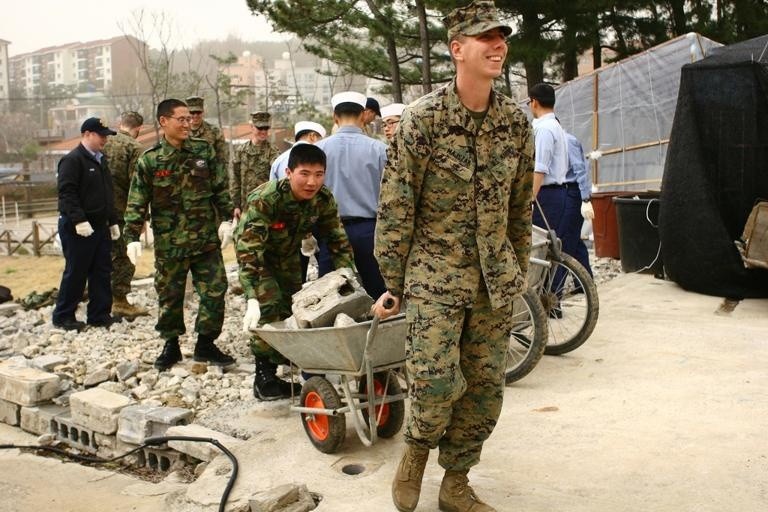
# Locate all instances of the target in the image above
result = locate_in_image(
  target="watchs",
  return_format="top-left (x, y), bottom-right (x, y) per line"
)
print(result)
top-left (581, 199), bottom-right (591, 203)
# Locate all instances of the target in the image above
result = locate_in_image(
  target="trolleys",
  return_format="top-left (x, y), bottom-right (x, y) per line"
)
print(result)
top-left (503, 223), bottom-right (602, 386)
top-left (250, 299), bottom-right (405, 454)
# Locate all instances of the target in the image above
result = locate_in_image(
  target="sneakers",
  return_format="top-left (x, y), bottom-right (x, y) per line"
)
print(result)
top-left (569, 287), bottom-right (584, 294)
top-left (548, 303), bottom-right (562, 319)
top-left (53, 318), bottom-right (85, 330)
top-left (87, 314), bottom-right (120, 325)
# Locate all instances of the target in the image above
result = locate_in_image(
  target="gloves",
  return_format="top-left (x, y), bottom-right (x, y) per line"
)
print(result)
top-left (242, 299), bottom-right (261, 336)
top-left (126, 241), bottom-right (141, 265)
top-left (110, 225), bottom-right (120, 240)
top-left (581, 200), bottom-right (595, 220)
top-left (218, 222), bottom-right (231, 250)
top-left (75, 221), bottom-right (94, 237)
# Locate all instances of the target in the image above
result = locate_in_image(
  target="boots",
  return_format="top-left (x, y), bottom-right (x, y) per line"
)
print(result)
top-left (193, 334), bottom-right (235, 367)
top-left (153, 338), bottom-right (183, 370)
top-left (438, 470), bottom-right (497, 512)
top-left (391, 444), bottom-right (429, 511)
top-left (231, 285), bottom-right (243, 294)
top-left (252, 356), bottom-right (302, 400)
top-left (113, 299), bottom-right (149, 319)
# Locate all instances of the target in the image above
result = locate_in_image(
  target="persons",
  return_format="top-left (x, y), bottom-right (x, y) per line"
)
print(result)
top-left (103, 111), bottom-right (150, 321)
top-left (229, 110), bottom-right (281, 295)
top-left (556, 116), bottom-right (597, 295)
top-left (314, 90), bottom-right (388, 305)
top-left (231, 144), bottom-right (361, 402)
top-left (121, 99), bottom-right (235, 373)
top-left (526, 83), bottom-right (568, 319)
top-left (51, 117), bottom-right (124, 331)
top-left (379, 102), bottom-right (406, 144)
top-left (186, 96), bottom-right (229, 184)
top-left (371, 1), bottom-right (537, 512)
top-left (330, 98), bottom-right (382, 137)
top-left (270, 121), bottom-right (335, 284)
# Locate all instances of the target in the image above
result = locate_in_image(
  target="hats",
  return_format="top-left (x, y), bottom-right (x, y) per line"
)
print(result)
top-left (330, 91), bottom-right (367, 110)
top-left (80, 117), bottom-right (118, 137)
top-left (185, 96), bottom-right (205, 113)
top-left (367, 97), bottom-right (381, 118)
top-left (249, 111), bottom-right (271, 128)
top-left (294, 120), bottom-right (327, 138)
top-left (120, 110), bottom-right (143, 124)
top-left (380, 102), bottom-right (406, 118)
top-left (441, 0), bottom-right (513, 39)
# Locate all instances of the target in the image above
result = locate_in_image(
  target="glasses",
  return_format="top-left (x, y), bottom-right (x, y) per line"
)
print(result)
top-left (256, 126), bottom-right (269, 131)
top-left (382, 120), bottom-right (398, 128)
top-left (525, 100), bottom-right (532, 106)
top-left (171, 116), bottom-right (193, 124)
top-left (189, 111), bottom-right (202, 115)
